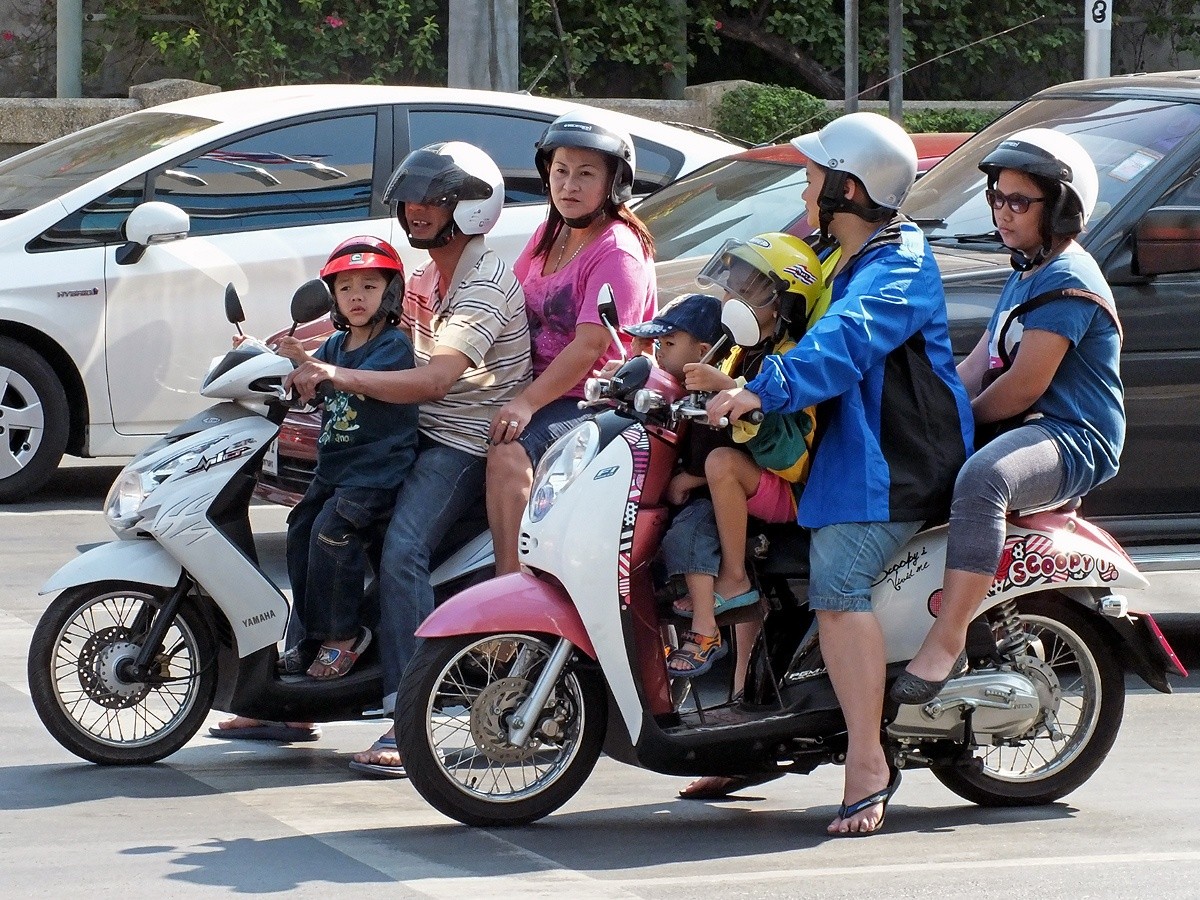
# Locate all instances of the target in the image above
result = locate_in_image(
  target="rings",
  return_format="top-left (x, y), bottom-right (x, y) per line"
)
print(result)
top-left (501, 421), bottom-right (507, 425)
top-left (511, 422), bottom-right (518, 427)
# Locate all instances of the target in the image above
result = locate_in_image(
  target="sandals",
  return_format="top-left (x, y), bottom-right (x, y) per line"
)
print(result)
top-left (665, 625), bottom-right (729, 677)
top-left (891, 646), bottom-right (970, 705)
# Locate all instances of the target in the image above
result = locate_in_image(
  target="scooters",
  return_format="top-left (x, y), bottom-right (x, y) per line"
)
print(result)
top-left (28, 280), bottom-right (498, 768)
top-left (393, 282), bottom-right (1187, 826)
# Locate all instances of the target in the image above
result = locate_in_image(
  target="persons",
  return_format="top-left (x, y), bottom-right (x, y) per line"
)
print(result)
top-left (890, 128), bottom-right (1127, 705)
top-left (594, 112), bottom-right (974, 838)
top-left (209, 110), bottom-right (660, 779)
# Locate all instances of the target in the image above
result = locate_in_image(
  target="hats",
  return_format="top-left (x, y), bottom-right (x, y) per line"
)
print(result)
top-left (622, 294), bottom-right (733, 356)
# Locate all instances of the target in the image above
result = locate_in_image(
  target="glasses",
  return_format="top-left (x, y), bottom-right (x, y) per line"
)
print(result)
top-left (985, 188), bottom-right (1047, 213)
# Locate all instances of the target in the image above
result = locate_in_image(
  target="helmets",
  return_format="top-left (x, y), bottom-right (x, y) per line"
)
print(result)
top-left (789, 112), bottom-right (919, 210)
top-left (320, 234), bottom-right (405, 302)
top-left (976, 128), bottom-right (1099, 234)
top-left (695, 232), bottom-right (822, 321)
top-left (379, 139), bottom-right (506, 238)
top-left (536, 110), bottom-right (635, 203)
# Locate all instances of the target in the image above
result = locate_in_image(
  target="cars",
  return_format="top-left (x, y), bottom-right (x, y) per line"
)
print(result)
top-left (651, 68), bottom-right (1200, 518)
top-left (257, 133), bottom-right (966, 509)
top-left (0, 85), bottom-right (750, 502)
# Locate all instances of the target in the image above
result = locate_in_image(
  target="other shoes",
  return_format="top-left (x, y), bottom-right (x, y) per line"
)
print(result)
top-left (465, 644), bottom-right (518, 678)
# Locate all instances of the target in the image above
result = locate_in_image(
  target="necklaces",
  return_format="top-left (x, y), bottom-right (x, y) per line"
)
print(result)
top-left (1031, 238), bottom-right (1065, 274)
top-left (553, 220), bottom-right (603, 273)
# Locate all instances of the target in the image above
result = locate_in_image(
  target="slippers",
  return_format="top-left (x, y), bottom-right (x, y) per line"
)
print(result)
top-left (671, 587), bottom-right (759, 619)
top-left (678, 770), bottom-right (786, 797)
top-left (208, 716), bottom-right (322, 741)
top-left (307, 625), bottom-right (374, 681)
top-left (277, 646), bottom-right (306, 675)
top-left (350, 734), bottom-right (446, 777)
top-left (826, 766), bottom-right (902, 836)
